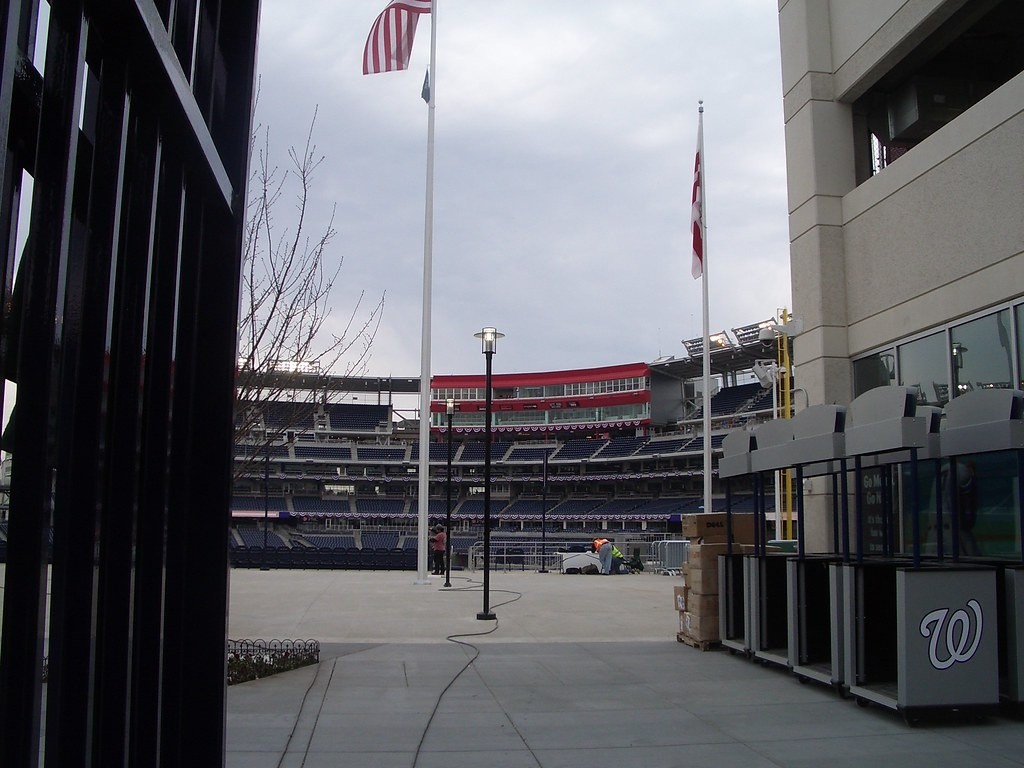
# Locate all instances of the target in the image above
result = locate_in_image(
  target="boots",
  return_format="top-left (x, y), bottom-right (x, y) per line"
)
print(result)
top-left (432, 569), bottom-right (439, 575)
top-left (440, 570), bottom-right (444, 575)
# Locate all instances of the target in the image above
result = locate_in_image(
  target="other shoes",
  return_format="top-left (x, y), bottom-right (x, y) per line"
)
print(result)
top-left (602, 573), bottom-right (609, 575)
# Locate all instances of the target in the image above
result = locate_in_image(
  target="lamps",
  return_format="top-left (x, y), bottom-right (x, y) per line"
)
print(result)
top-left (753, 358), bottom-right (786, 390)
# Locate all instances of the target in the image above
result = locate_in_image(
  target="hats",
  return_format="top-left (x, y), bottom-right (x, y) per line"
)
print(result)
top-left (592, 537), bottom-right (598, 542)
top-left (433, 524), bottom-right (442, 530)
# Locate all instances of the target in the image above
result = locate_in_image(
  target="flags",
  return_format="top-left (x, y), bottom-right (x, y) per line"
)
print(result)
top-left (690, 115), bottom-right (705, 280)
top-left (363, 0), bottom-right (436, 75)
top-left (421, 68), bottom-right (433, 104)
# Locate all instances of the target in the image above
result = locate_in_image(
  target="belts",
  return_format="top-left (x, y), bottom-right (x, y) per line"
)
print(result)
top-left (602, 542), bottom-right (609, 545)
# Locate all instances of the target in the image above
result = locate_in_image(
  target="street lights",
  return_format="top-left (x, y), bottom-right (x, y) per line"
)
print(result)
top-left (437, 398), bottom-right (461, 587)
top-left (473, 326), bottom-right (505, 620)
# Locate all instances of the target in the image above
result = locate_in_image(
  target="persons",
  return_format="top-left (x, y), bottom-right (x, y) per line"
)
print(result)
top-left (429, 524), bottom-right (447, 575)
top-left (610, 545), bottom-right (624, 575)
top-left (591, 537), bottom-right (612, 575)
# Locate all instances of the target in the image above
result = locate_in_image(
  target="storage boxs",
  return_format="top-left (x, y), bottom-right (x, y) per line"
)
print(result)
top-left (673, 512), bottom-right (762, 644)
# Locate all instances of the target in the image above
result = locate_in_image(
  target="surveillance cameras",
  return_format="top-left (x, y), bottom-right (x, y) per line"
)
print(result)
top-left (759, 329), bottom-right (775, 347)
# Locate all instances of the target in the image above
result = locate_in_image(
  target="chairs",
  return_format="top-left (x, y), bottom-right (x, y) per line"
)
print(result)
top-left (495, 548), bottom-right (526, 572)
top-left (235, 546), bottom-right (418, 572)
